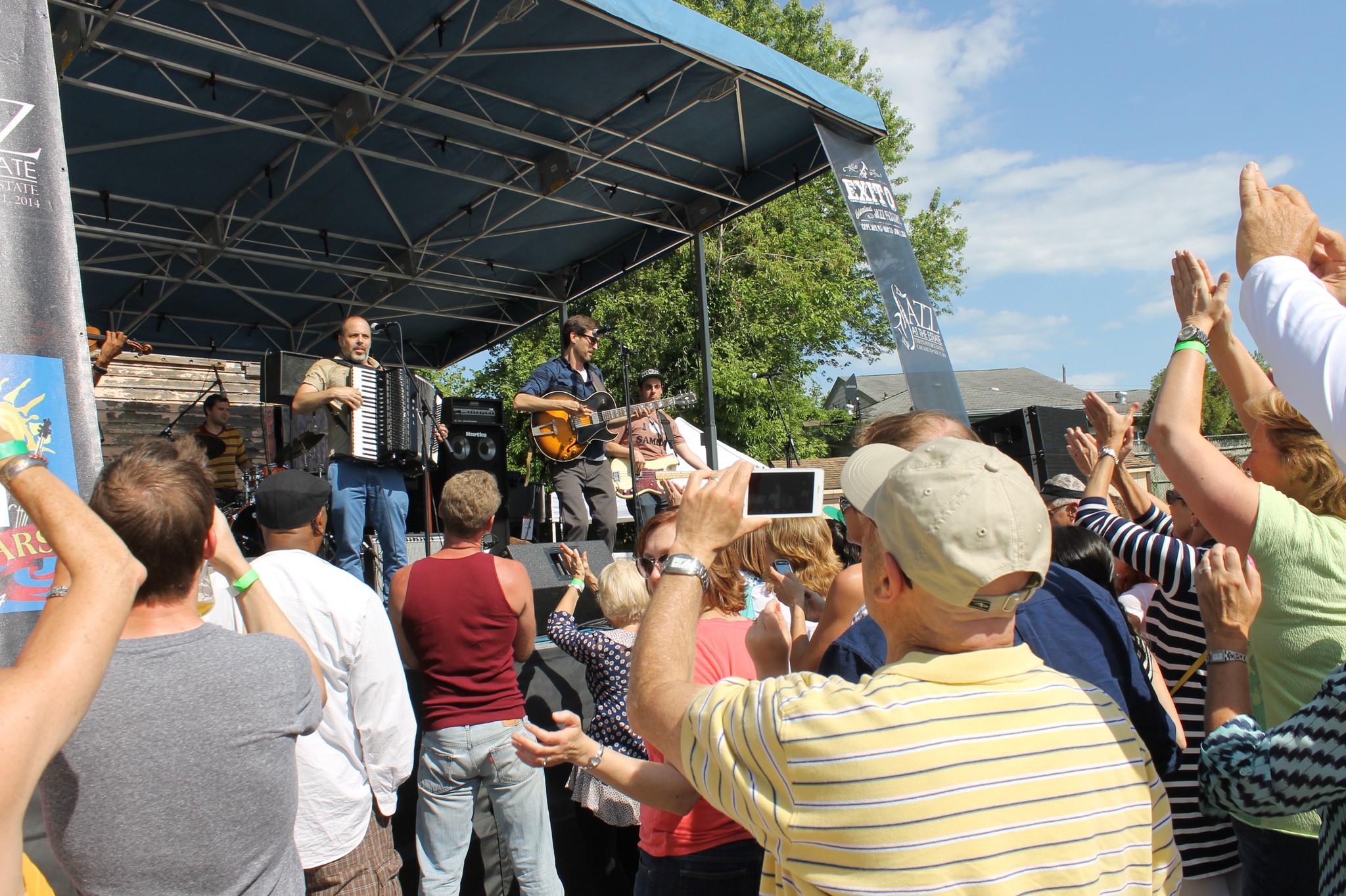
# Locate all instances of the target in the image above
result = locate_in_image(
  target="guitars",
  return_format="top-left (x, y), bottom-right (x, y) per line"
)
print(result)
top-left (531, 388), bottom-right (698, 465)
top-left (610, 456), bottom-right (718, 500)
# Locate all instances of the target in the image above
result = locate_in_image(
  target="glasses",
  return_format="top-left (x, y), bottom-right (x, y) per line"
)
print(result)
top-left (635, 554), bottom-right (668, 580)
top-left (839, 496), bottom-right (878, 529)
top-left (1165, 489), bottom-right (1184, 505)
top-left (575, 333), bottom-right (600, 347)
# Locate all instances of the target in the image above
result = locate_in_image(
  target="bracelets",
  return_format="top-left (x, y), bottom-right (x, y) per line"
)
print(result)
top-left (225, 567), bottom-right (259, 598)
top-left (46, 586), bottom-right (70, 600)
top-left (570, 578), bottom-right (585, 590)
top-left (0, 454), bottom-right (48, 490)
top-left (567, 584), bottom-right (584, 594)
top-left (0, 441), bottom-right (30, 460)
top-left (1171, 341), bottom-right (1206, 356)
top-left (92, 363), bottom-right (109, 376)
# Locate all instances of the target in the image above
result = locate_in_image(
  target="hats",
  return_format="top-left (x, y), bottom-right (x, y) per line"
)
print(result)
top-left (841, 436), bottom-right (1052, 611)
top-left (1039, 484), bottom-right (1084, 501)
top-left (823, 506), bottom-right (846, 526)
top-left (637, 368), bottom-right (664, 387)
top-left (254, 469), bottom-right (332, 529)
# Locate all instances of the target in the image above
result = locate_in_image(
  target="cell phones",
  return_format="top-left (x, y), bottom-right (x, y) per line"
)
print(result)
top-left (741, 468), bottom-right (825, 519)
top-left (773, 559), bottom-right (794, 576)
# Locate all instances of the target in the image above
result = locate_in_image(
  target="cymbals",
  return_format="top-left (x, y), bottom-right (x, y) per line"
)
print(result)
top-left (274, 431), bottom-right (326, 463)
top-left (304, 471), bottom-right (328, 476)
top-left (170, 435), bottom-right (227, 460)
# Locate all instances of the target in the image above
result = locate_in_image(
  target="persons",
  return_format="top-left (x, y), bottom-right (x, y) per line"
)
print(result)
top-left (0, 165), bottom-right (1346, 896)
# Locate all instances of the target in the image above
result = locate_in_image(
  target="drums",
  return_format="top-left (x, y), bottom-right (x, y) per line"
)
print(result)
top-left (223, 504), bottom-right (267, 559)
top-left (246, 464), bottom-right (288, 496)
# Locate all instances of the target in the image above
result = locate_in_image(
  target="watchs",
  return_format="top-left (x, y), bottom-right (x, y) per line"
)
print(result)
top-left (660, 554), bottom-right (709, 596)
top-left (1174, 324), bottom-right (1211, 350)
top-left (1098, 446), bottom-right (1119, 464)
top-left (578, 743), bottom-right (604, 770)
top-left (1205, 649), bottom-right (1247, 663)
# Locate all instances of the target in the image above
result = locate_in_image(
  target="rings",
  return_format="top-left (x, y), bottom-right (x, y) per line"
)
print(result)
top-left (753, 617), bottom-right (758, 622)
top-left (543, 755), bottom-right (547, 767)
top-left (711, 477), bottom-right (720, 483)
top-left (1074, 449), bottom-right (1080, 456)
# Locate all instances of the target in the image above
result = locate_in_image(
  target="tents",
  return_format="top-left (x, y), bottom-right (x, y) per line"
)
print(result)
top-left (551, 416), bottom-right (767, 544)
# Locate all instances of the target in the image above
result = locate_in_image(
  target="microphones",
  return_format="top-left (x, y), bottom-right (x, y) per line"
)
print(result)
top-left (592, 326), bottom-right (618, 337)
top-left (213, 365), bottom-right (227, 398)
top-left (370, 322), bottom-right (398, 334)
top-left (751, 372), bottom-right (784, 380)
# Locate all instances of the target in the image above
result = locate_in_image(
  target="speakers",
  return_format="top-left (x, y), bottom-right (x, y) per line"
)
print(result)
top-left (429, 426), bottom-right (509, 558)
top-left (971, 405), bottom-right (1089, 494)
top-left (364, 532), bottom-right (444, 606)
top-left (259, 351), bottom-right (323, 405)
top-left (496, 539), bottom-right (614, 636)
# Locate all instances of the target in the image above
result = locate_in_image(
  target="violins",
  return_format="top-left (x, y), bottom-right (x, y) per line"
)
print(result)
top-left (86, 326), bottom-right (152, 356)
